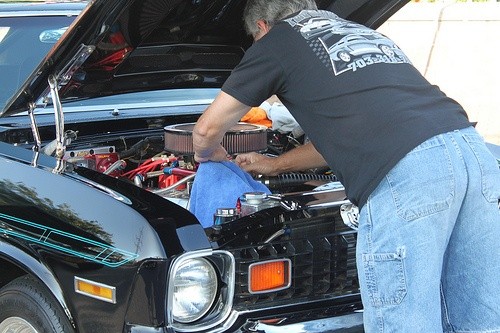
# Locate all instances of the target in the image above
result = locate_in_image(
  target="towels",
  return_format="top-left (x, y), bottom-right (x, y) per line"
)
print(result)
top-left (186, 157), bottom-right (275, 230)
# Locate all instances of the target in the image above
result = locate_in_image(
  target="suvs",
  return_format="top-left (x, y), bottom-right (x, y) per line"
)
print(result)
top-left (1, 1), bottom-right (500, 333)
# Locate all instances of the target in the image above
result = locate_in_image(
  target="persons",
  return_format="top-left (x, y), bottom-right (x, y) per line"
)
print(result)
top-left (193, 0), bottom-right (500, 333)
